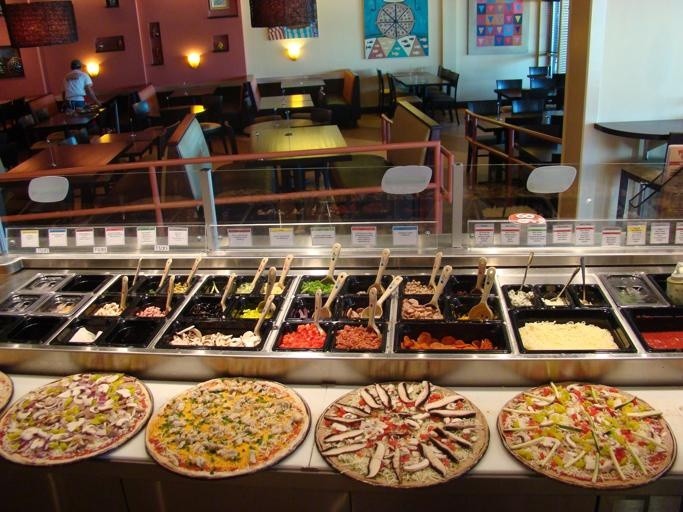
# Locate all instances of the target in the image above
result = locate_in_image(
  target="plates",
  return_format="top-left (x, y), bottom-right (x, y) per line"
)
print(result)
top-left (89, 104), bottom-right (98, 109)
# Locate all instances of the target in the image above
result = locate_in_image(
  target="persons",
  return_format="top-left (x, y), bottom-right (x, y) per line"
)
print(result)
top-left (60, 59), bottom-right (103, 114)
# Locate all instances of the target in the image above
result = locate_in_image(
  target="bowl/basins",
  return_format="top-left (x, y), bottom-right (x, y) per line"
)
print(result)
top-left (80, 109), bottom-right (87, 115)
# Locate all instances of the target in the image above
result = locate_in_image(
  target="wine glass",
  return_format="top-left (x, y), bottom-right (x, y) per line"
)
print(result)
top-left (181, 80), bottom-right (189, 94)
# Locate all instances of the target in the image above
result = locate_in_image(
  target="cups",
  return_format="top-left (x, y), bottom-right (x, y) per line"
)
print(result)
top-left (184, 86), bottom-right (192, 96)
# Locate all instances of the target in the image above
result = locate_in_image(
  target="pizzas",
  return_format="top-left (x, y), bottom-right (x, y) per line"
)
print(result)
top-left (0, 371), bottom-right (15, 416)
top-left (317, 380), bottom-right (487, 488)
top-left (499, 379), bottom-right (674, 491)
top-left (145, 376), bottom-right (311, 480)
top-left (0, 371), bottom-right (152, 466)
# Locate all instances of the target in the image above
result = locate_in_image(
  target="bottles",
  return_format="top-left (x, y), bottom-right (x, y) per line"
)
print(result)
top-left (45, 137), bottom-right (59, 168)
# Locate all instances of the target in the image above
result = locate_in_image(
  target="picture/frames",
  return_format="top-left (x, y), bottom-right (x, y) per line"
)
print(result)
top-left (207, 0), bottom-right (238, 18)
top-left (2, 0), bottom-right (78, 48)
top-left (0, 46), bottom-right (25, 79)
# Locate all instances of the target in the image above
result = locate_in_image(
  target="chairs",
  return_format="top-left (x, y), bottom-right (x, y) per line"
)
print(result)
top-left (0, 66), bottom-right (683, 224)
